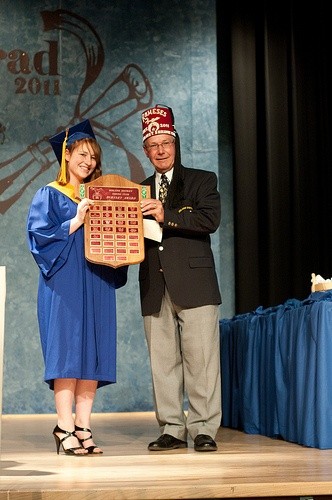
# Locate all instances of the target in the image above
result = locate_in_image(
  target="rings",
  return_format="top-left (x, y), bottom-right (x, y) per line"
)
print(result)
top-left (155, 203), bottom-right (156, 208)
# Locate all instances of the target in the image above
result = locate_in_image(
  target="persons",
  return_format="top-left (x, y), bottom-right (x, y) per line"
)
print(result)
top-left (137, 106), bottom-right (221, 451)
top-left (28, 119), bottom-right (128, 456)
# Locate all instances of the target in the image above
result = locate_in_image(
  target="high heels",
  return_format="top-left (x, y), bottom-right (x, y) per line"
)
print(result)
top-left (75, 425), bottom-right (103, 454)
top-left (53, 425), bottom-right (88, 455)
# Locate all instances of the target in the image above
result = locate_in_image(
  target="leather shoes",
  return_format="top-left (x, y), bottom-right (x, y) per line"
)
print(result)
top-left (194, 434), bottom-right (217, 450)
top-left (148, 434), bottom-right (188, 450)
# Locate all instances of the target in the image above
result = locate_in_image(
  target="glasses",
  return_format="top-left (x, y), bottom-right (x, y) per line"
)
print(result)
top-left (145, 139), bottom-right (175, 152)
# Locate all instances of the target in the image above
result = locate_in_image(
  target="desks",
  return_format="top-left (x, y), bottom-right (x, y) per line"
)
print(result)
top-left (219, 289), bottom-right (332, 450)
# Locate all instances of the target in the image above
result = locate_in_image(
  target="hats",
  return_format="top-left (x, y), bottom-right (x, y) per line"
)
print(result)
top-left (48, 119), bottom-right (97, 184)
top-left (141, 104), bottom-right (177, 143)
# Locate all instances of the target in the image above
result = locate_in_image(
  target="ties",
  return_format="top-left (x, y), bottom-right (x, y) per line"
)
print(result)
top-left (158, 174), bottom-right (170, 209)
